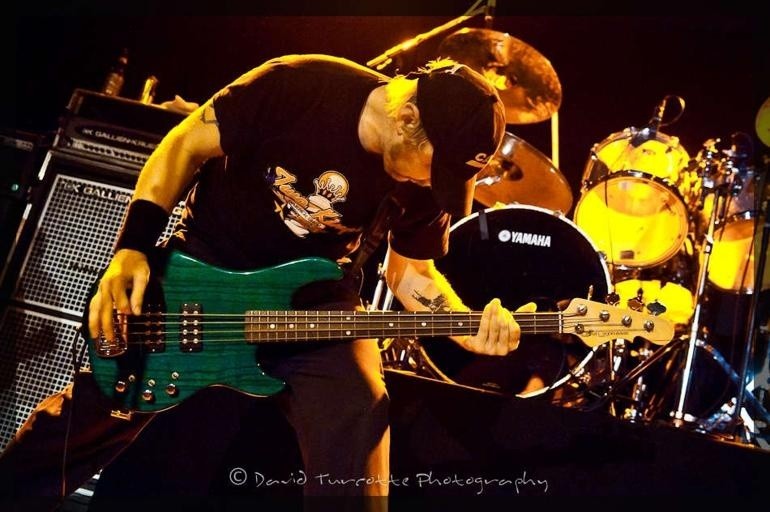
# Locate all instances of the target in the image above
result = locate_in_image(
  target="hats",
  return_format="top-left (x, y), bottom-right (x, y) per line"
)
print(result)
top-left (418, 62), bottom-right (508, 216)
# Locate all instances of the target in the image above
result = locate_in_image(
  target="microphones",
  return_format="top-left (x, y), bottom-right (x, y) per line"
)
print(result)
top-left (648, 102), bottom-right (665, 138)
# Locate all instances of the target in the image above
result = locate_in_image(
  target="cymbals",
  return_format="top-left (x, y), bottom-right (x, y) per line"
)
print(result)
top-left (438, 25), bottom-right (562, 125)
top-left (474, 132), bottom-right (575, 216)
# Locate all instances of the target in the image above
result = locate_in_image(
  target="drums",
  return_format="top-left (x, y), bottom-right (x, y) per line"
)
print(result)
top-left (694, 169), bottom-right (765, 294)
top-left (413, 204), bottom-right (615, 400)
top-left (574, 127), bottom-right (703, 271)
top-left (607, 237), bottom-right (700, 329)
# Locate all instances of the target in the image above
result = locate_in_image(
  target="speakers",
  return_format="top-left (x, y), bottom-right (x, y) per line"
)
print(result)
top-left (0, 88), bottom-right (198, 499)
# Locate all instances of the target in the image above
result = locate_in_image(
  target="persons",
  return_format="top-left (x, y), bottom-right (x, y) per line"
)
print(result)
top-left (1, 51), bottom-right (539, 510)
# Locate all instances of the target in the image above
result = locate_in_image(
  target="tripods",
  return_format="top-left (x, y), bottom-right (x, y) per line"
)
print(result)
top-left (585, 161), bottom-right (770, 448)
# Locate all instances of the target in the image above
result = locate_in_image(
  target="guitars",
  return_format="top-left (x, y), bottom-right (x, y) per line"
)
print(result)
top-left (82, 246), bottom-right (675, 414)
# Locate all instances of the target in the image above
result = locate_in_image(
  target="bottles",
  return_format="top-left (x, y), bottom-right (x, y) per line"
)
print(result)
top-left (102, 57), bottom-right (129, 97)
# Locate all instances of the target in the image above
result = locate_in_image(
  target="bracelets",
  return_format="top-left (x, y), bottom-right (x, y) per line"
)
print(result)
top-left (111, 197), bottom-right (170, 259)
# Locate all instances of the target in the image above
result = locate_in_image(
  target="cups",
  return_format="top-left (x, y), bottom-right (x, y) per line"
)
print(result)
top-left (139, 77), bottom-right (160, 105)
